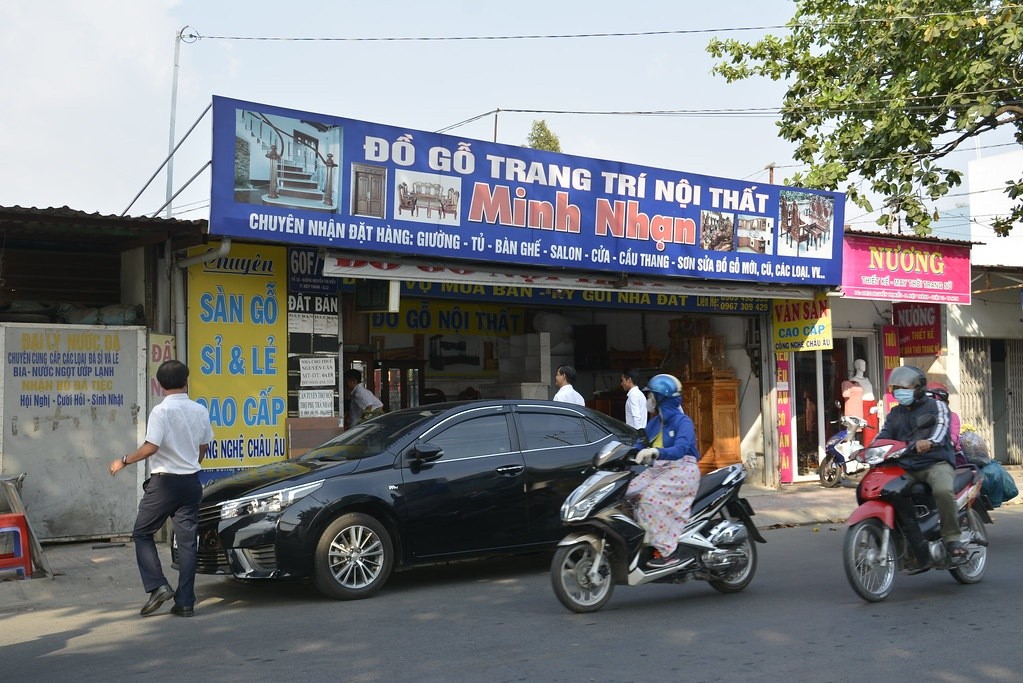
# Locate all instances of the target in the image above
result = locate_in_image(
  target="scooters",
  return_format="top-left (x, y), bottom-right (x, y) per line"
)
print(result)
top-left (551, 438), bottom-right (768, 611)
top-left (819, 414), bottom-right (877, 488)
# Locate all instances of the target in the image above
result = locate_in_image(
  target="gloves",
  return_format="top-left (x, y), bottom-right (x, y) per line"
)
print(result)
top-left (636, 447), bottom-right (659, 465)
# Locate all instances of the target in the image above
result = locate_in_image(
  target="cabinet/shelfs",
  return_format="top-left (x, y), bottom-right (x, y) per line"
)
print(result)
top-left (496, 331), bottom-right (552, 385)
top-left (342, 350), bottom-right (375, 402)
top-left (351, 161), bottom-right (386, 219)
top-left (690, 333), bottom-right (727, 373)
top-left (375, 359), bottom-right (428, 413)
top-left (737, 218), bottom-right (767, 253)
top-left (668, 314), bottom-right (713, 380)
top-left (478, 382), bottom-right (549, 400)
top-left (684, 379), bottom-right (742, 477)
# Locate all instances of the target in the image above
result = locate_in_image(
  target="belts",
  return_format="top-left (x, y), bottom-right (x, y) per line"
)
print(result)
top-left (151, 472), bottom-right (166, 475)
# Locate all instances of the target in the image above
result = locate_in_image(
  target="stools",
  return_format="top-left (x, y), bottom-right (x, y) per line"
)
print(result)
top-left (0, 512), bottom-right (33, 581)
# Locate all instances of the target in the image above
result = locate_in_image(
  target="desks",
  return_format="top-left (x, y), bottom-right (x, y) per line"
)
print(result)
top-left (413, 193), bottom-right (446, 220)
top-left (798, 213), bottom-right (817, 250)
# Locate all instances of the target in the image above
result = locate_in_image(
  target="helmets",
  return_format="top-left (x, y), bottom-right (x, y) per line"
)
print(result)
top-left (641, 374), bottom-right (682, 397)
top-left (926, 380), bottom-right (949, 402)
top-left (892, 365), bottom-right (926, 399)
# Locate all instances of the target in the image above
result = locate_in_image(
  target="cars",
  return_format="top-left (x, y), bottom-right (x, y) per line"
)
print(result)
top-left (168, 399), bottom-right (637, 599)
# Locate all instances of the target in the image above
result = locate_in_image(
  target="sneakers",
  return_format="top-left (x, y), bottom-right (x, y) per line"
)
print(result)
top-left (646, 550), bottom-right (680, 566)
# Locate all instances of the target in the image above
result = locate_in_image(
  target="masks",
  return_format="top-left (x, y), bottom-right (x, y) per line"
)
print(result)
top-left (646, 399), bottom-right (657, 413)
top-left (893, 388), bottom-right (915, 405)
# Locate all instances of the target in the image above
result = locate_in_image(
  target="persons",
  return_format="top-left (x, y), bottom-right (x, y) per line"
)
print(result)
top-left (842, 380), bottom-right (864, 441)
top-left (850, 359), bottom-right (879, 458)
top-left (553, 366), bottom-right (585, 407)
top-left (924, 381), bottom-right (969, 466)
top-left (345, 369), bottom-right (383, 429)
top-left (621, 366), bottom-right (648, 430)
top-left (108, 360), bottom-right (215, 617)
top-left (849, 364), bottom-right (969, 556)
top-left (624, 374), bottom-right (701, 567)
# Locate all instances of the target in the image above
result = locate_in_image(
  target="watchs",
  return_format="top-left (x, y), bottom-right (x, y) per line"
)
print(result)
top-left (122, 455), bottom-right (130, 465)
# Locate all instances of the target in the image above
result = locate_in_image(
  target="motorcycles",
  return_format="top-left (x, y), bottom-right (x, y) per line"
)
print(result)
top-left (836, 414), bottom-right (995, 600)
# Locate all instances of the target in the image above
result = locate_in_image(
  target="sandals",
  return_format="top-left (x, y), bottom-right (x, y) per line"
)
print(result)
top-left (947, 541), bottom-right (969, 556)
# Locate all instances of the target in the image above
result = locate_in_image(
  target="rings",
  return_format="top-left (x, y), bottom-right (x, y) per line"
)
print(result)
top-left (111, 468), bottom-right (114, 471)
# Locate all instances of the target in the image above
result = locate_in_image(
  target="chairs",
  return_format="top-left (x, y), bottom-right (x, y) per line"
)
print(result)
top-left (420, 388), bottom-right (447, 404)
top-left (780, 193), bottom-right (833, 256)
top-left (457, 387), bottom-right (482, 399)
top-left (397, 181), bottom-right (459, 220)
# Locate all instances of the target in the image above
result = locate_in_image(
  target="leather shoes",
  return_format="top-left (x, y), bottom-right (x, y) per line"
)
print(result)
top-left (170, 603), bottom-right (193, 617)
top-left (140, 584), bottom-right (173, 614)
